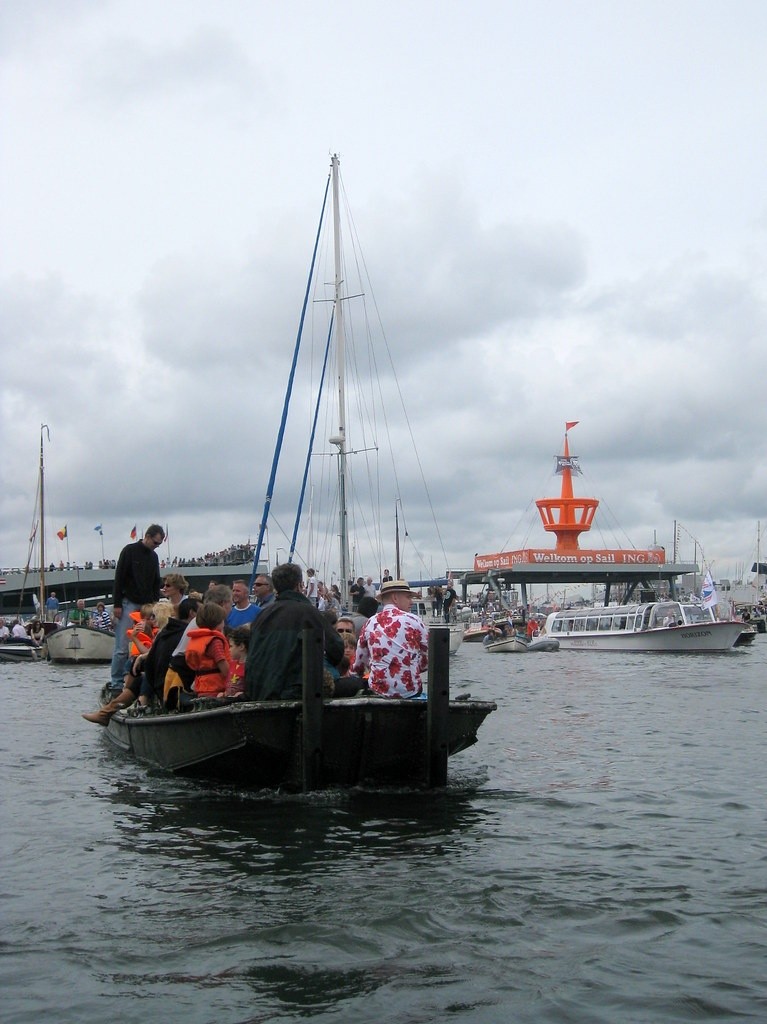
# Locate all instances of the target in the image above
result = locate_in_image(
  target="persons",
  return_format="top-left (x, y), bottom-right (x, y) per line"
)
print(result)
top-left (110, 525), bottom-right (165, 697)
top-left (0, 592), bottom-right (118, 644)
top-left (82, 524), bottom-right (766, 726)
top-left (244, 564), bottom-right (344, 700)
top-left (352, 580), bottom-right (429, 701)
top-left (0, 540), bottom-right (254, 575)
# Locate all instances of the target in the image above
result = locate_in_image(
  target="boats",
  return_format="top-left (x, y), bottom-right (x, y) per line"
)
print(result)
top-left (0, 155), bottom-right (767, 665)
top-left (101, 627), bottom-right (498, 791)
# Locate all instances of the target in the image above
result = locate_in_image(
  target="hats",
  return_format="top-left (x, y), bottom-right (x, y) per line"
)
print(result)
top-left (376, 581), bottom-right (416, 602)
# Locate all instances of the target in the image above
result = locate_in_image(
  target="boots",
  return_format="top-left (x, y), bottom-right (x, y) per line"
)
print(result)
top-left (82, 688), bottom-right (137, 726)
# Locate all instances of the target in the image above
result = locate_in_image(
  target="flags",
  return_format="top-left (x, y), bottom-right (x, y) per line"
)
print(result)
top-left (701, 571), bottom-right (719, 611)
top-left (94, 526), bottom-right (102, 535)
top-left (130, 526), bottom-right (136, 539)
top-left (56, 527), bottom-right (67, 540)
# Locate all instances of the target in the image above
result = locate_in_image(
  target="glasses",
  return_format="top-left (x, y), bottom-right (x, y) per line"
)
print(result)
top-left (165, 584), bottom-right (174, 588)
top-left (253, 583), bottom-right (269, 587)
top-left (150, 536), bottom-right (161, 545)
top-left (36, 624), bottom-right (40, 626)
top-left (337, 629), bottom-right (354, 633)
